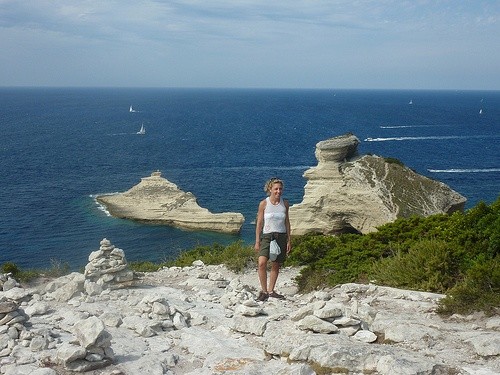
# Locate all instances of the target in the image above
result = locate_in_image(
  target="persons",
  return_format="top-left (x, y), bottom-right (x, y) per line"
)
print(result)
top-left (254, 177), bottom-right (292, 301)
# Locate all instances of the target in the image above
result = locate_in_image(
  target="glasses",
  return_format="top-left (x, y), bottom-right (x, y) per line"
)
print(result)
top-left (269, 178), bottom-right (281, 185)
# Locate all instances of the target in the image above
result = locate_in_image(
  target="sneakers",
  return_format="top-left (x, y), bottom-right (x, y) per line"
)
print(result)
top-left (267, 291), bottom-right (284, 300)
top-left (255, 291), bottom-right (269, 302)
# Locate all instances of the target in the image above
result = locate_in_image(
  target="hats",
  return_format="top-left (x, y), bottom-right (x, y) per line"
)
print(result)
top-left (269, 239), bottom-right (281, 261)
